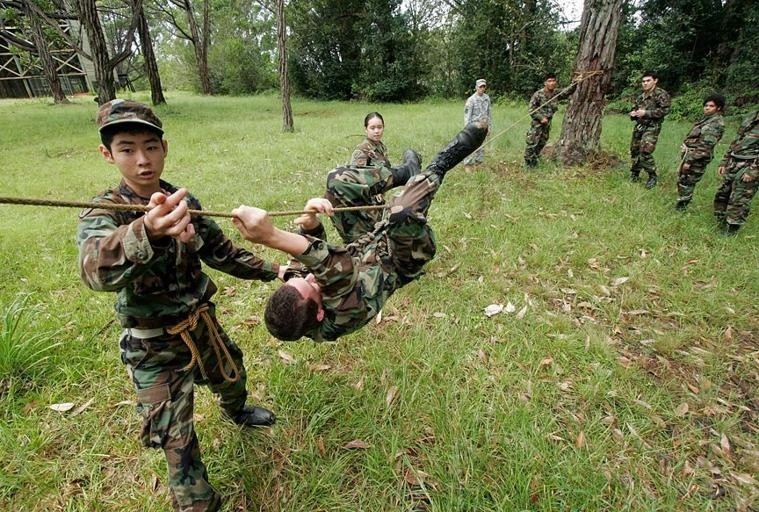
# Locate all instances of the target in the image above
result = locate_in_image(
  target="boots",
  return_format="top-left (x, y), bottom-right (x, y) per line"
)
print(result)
top-left (389, 149), bottom-right (422, 187)
top-left (645, 172), bottom-right (657, 189)
top-left (427, 120), bottom-right (488, 184)
top-left (219, 398), bottom-right (275, 426)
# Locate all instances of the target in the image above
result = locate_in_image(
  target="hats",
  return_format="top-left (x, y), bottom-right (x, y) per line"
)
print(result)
top-left (95, 100), bottom-right (166, 137)
top-left (475, 79), bottom-right (487, 88)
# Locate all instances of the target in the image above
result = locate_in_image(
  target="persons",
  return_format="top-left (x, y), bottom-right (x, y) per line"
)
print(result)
top-left (713, 108), bottom-right (758, 231)
top-left (463, 78), bottom-right (491, 175)
top-left (228, 120), bottom-right (489, 341)
top-left (628, 73), bottom-right (670, 188)
top-left (524, 75), bottom-right (581, 167)
top-left (348, 112), bottom-right (390, 165)
top-left (675, 94), bottom-right (726, 209)
top-left (77, 99), bottom-right (296, 511)
